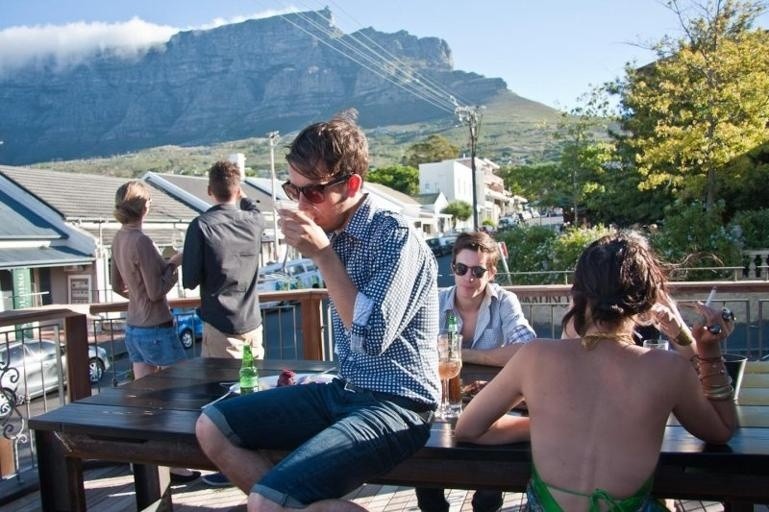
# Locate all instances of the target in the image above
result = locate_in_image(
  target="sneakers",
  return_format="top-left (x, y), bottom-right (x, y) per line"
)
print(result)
top-left (202, 473), bottom-right (234, 486)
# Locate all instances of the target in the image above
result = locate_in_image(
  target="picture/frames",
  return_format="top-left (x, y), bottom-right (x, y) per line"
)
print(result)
top-left (66, 272), bottom-right (93, 305)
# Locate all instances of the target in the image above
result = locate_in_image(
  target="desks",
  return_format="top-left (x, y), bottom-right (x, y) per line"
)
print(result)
top-left (29, 355), bottom-right (768, 512)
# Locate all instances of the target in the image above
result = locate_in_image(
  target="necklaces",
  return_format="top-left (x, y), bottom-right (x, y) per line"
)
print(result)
top-left (584, 332), bottom-right (635, 345)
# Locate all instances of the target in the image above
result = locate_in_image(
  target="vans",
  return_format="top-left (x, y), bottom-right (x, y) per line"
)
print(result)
top-left (256, 258), bottom-right (324, 312)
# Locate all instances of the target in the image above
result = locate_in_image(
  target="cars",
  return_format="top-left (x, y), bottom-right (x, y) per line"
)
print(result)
top-left (426, 216), bottom-right (516, 257)
top-left (0, 337), bottom-right (111, 418)
top-left (168, 302), bottom-right (204, 348)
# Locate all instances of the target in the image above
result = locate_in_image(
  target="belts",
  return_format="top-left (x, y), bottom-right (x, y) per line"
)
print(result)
top-left (343, 382), bottom-right (363, 393)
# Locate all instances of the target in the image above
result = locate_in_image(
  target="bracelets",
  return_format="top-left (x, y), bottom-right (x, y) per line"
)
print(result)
top-left (673, 326), bottom-right (693, 346)
top-left (696, 356), bottom-right (735, 401)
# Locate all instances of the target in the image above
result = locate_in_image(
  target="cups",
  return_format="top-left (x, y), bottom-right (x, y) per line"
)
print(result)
top-left (643, 339), bottom-right (668, 352)
top-left (721, 352), bottom-right (749, 402)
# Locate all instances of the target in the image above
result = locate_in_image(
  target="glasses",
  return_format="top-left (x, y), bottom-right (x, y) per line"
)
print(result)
top-left (450, 263), bottom-right (488, 279)
top-left (281, 174), bottom-right (349, 203)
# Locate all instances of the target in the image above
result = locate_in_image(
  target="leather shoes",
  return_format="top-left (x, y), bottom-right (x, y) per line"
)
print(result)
top-left (170, 471), bottom-right (201, 483)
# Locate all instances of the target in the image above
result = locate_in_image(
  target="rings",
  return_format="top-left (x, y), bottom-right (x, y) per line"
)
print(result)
top-left (708, 325), bottom-right (722, 335)
top-left (721, 307), bottom-right (735, 322)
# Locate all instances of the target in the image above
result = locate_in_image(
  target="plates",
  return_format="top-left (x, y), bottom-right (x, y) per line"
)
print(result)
top-left (228, 372), bottom-right (340, 395)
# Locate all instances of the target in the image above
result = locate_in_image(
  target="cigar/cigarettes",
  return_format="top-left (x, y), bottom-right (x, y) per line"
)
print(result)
top-left (705, 286), bottom-right (718, 307)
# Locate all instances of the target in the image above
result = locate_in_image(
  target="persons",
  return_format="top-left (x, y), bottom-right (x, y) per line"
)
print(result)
top-left (195, 119), bottom-right (442, 512)
top-left (438, 232), bottom-right (537, 367)
top-left (110, 181), bottom-right (200, 482)
top-left (456, 303), bottom-right (740, 511)
top-left (741, 253), bottom-right (769, 278)
top-left (560, 230), bottom-right (698, 359)
top-left (183, 162), bottom-right (265, 487)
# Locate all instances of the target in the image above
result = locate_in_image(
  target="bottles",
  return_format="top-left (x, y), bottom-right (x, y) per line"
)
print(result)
top-left (237, 344), bottom-right (259, 394)
top-left (447, 314), bottom-right (461, 402)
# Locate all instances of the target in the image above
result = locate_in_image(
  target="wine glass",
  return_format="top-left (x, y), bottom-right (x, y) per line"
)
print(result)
top-left (270, 200), bottom-right (323, 263)
top-left (435, 334), bottom-right (463, 421)
top-left (171, 233), bottom-right (186, 254)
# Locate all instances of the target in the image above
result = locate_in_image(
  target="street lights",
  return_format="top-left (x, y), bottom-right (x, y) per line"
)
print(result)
top-left (267, 131), bottom-right (280, 261)
